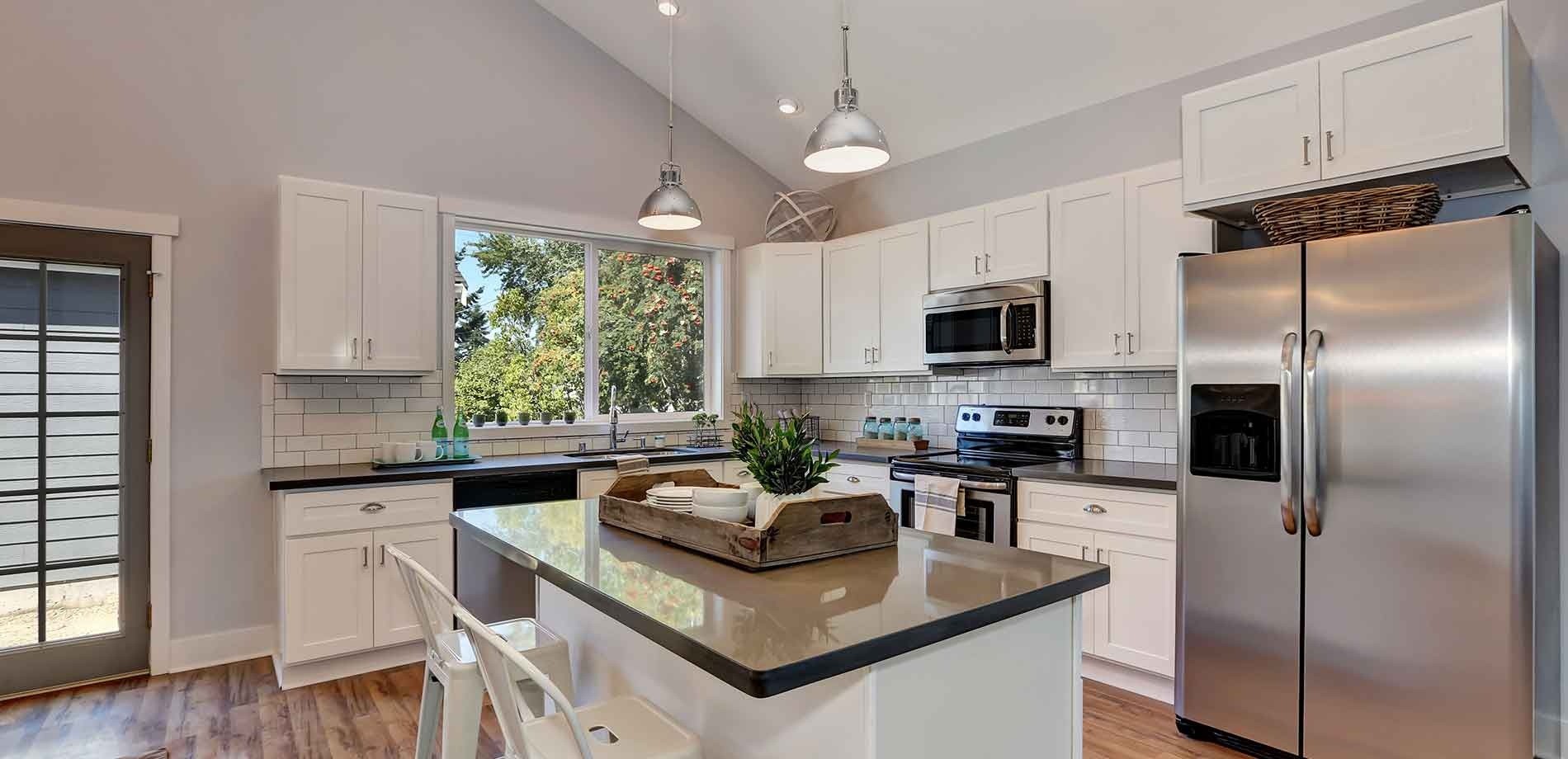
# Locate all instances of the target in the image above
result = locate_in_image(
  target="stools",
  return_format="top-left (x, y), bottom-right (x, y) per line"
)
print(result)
top-left (384, 544), bottom-right (703, 759)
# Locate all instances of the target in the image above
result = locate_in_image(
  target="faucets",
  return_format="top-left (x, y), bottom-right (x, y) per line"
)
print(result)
top-left (609, 384), bottom-right (629, 449)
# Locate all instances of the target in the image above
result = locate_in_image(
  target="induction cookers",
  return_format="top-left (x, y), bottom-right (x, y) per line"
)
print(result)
top-left (890, 404), bottom-right (1084, 479)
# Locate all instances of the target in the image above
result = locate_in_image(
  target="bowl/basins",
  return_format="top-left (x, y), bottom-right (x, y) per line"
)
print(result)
top-left (692, 503), bottom-right (748, 523)
top-left (911, 439), bottom-right (930, 450)
top-left (692, 488), bottom-right (748, 507)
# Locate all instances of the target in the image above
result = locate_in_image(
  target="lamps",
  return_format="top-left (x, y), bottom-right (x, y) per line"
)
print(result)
top-left (638, 1), bottom-right (703, 230)
top-left (803, 1), bottom-right (891, 174)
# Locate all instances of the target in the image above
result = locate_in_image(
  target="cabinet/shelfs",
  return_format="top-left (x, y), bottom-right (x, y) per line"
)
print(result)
top-left (268, 478), bottom-right (455, 691)
top-left (736, 157), bottom-right (1244, 379)
top-left (1015, 477), bottom-right (1176, 705)
top-left (578, 456), bottom-right (890, 513)
top-left (266, 175), bottom-right (438, 378)
top-left (1180, 2), bottom-right (1533, 229)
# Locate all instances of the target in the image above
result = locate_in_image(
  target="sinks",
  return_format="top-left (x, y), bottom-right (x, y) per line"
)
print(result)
top-left (562, 447), bottom-right (699, 460)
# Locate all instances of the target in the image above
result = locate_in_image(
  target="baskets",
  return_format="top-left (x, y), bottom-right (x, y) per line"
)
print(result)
top-left (1251, 182), bottom-right (1444, 247)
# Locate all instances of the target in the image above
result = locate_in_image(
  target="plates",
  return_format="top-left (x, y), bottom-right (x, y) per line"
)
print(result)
top-left (646, 486), bottom-right (704, 513)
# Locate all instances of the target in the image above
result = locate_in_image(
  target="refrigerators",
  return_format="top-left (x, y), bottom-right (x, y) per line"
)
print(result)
top-left (1172, 203), bottom-right (1562, 759)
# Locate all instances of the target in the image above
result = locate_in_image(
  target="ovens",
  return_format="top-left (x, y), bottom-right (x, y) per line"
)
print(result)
top-left (888, 467), bottom-right (1017, 548)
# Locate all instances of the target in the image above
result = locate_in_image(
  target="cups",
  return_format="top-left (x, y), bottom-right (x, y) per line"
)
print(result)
top-left (395, 443), bottom-right (423, 463)
top-left (541, 412), bottom-right (552, 425)
top-left (519, 413), bottom-right (531, 426)
top-left (740, 482), bottom-right (764, 520)
top-left (382, 441), bottom-right (396, 463)
top-left (563, 412), bottom-right (576, 424)
top-left (473, 415), bottom-right (485, 428)
top-left (416, 441), bottom-right (444, 462)
top-left (496, 412), bottom-right (508, 426)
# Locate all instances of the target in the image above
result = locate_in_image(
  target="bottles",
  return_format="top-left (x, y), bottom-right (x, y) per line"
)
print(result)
top-left (893, 417), bottom-right (908, 441)
top-left (654, 436), bottom-right (667, 448)
top-left (906, 417), bottom-right (922, 442)
top-left (453, 406), bottom-right (470, 458)
top-left (862, 416), bottom-right (879, 439)
top-left (877, 417), bottom-right (893, 440)
top-left (431, 404), bottom-right (449, 460)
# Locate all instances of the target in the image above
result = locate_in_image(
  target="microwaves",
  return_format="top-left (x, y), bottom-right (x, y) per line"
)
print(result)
top-left (921, 277), bottom-right (1050, 369)
